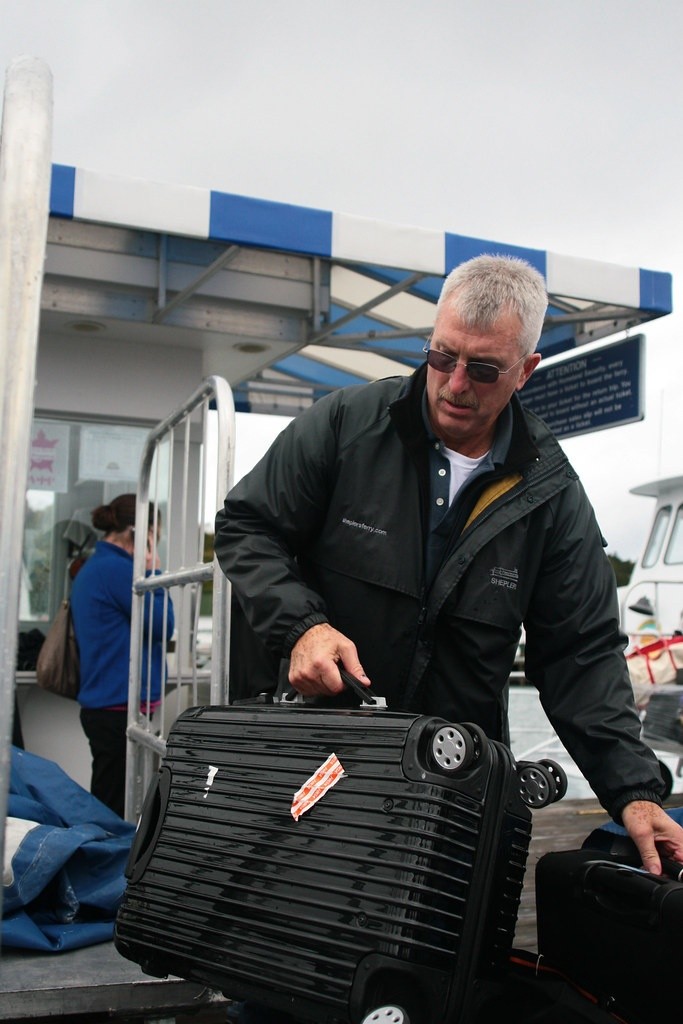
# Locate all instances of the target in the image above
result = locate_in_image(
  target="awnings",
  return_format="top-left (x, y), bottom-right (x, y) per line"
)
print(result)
top-left (205, 190), bottom-right (673, 420)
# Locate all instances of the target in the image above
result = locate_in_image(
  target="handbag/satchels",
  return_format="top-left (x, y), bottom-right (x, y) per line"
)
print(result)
top-left (37, 558), bottom-right (80, 699)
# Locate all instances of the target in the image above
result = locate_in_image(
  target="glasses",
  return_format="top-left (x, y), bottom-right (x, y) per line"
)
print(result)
top-left (422, 330), bottom-right (530, 385)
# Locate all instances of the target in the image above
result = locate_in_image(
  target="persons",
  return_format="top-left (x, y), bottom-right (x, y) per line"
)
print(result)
top-left (68, 493), bottom-right (174, 821)
top-left (209, 255), bottom-right (683, 876)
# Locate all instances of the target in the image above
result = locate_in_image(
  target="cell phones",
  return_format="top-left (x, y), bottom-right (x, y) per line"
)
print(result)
top-left (131, 527), bottom-right (151, 553)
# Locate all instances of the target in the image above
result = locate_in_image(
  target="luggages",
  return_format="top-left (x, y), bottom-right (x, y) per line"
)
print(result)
top-left (115, 660), bottom-right (568, 1023)
top-left (534, 822), bottom-right (683, 1024)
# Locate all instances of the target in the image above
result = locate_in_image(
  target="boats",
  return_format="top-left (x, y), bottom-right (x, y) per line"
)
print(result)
top-left (616, 476), bottom-right (683, 711)
